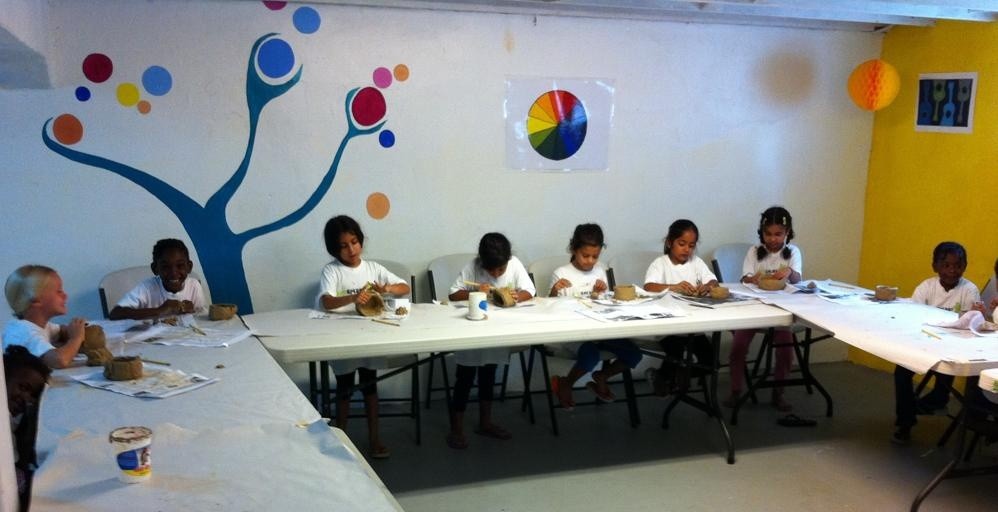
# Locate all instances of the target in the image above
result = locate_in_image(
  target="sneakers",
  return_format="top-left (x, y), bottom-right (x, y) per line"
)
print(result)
top-left (650, 369), bottom-right (670, 397)
top-left (920, 392), bottom-right (948, 412)
top-left (772, 392), bottom-right (793, 412)
top-left (727, 391), bottom-right (740, 409)
top-left (892, 425), bottom-right (911, 444)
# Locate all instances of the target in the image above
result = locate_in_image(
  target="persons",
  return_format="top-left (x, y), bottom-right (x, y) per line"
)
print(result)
top-left (722, 207), bottom-right (802, 410)
top-left (108, 238), bottom-right (206, 321)
top-left (547, 223), bottom-right (642, 412)
top-left (644, 219), bottom-right (717, 397)
top-left (890, 242), bottom-right (980, 449)
top-left (3, 264), bottom-right (86, 472)
top-left (963, 259), bottom-right (998, 445)
top-left (315, 214), bottom-right (409, 459)
top-left (446, 233), bottom-right (537, 447)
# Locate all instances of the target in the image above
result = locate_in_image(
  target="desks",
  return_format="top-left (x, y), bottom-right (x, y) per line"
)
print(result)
top-left (978, 368), bottom-right (998, 393)
top-left (792, 279), bottom-right (998, 512)
top-left (240, 292), bottom-right (794, 466)
top-left (28, 309), bottom-right (406, 512)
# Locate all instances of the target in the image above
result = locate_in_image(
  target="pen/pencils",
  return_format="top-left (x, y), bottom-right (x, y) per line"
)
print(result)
top-left (829, 283), bottom-right (855, 289)
top-left (372, 319), bottom-right (400, 326)
top-left (689, 303), bottom-right (714, 309)
top-left (921, 329), bottom-right (942, 339)
top-left (189, 324), bottom-right (205, 336)
top-left (464, 281), bottom-right (496, 290)
top-left (141, 358), bottom-right (171, 366)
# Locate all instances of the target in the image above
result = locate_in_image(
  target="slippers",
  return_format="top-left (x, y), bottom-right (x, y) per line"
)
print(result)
top-left (480, 426), bottom-right (511, 438)
top-left (372, 444), bottom-right (390, 458)
top-left (551, 375), bottom-right (575, 410)
top-left (445, 431), bottom-right (469, 450)
top-left (586, 379), bottom-right (616, 403)
top-left (776, 413), bottom-right (817, 428)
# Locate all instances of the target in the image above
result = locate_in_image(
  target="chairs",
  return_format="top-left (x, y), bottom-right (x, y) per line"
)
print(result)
top-left (710, 242), bottom-right (812, 407)
top-left (609, 251), bottom-right (713, 417)
top-left (309, 260), bottom-right (422, 446)
top-left (427, 254), bottom-right (537, 433)
top-left (894, 273), bottom-right (997, 460)
top-left (99, 265), bottom-right (202, 319)
top-left (528, 254), bottom-right (641, 437)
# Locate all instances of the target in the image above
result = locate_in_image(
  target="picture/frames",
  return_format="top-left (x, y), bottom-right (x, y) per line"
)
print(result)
top-left (914, 71), bottom-right (978, 135)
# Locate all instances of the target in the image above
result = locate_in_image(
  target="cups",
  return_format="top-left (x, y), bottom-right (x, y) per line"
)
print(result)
top-left (466, 292), bottom-right (488, 321)
top-left (109, 426), bottom-right (152, 484)
top-left (386, 298), bottom-right (410, 317)
